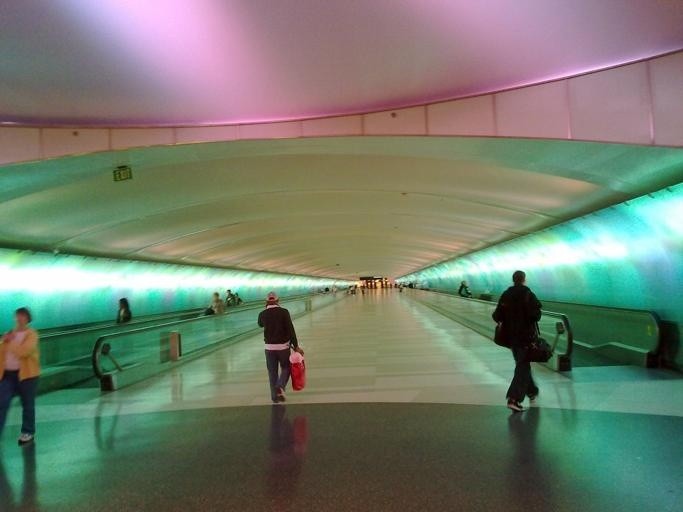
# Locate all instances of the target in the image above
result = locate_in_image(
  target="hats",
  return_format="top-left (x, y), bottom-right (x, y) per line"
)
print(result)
top-left (266, 291), bottom-right (279, 302)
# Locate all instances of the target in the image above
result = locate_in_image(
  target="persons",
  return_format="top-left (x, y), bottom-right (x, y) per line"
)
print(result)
top-left (224, 290), bottom-right (235, 306)
top-left (209, 292), bottom-right (224, 314)
top-left (257, 292), bottom-right (304, 403)
top-left (347, 283), bottom-right (368, 296)
top-left (331, 285), bottom-right (337, 299)
top-left (458, 281), bottom-right (472, 298)
top-left (115, 298), bottom-right (131, 323)
top-left (0, 306), bottom-right (40, 444)
top-left (491, 270), bottom-right (543, 412)
top-left (233, 293), bottom-right (243, 306)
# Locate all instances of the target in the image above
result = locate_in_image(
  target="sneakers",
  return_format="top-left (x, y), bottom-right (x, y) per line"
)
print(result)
top-left (507, 398), bottom-right (525, 413)
top-left (18, 433), bottom-right (34, 443)
top-left (529, 386), bottom-right (540, 403)
top-left (272, 386), bottom-right (286, 403)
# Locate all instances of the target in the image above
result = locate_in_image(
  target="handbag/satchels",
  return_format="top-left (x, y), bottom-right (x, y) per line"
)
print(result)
top-left (493, 323), bottom-right (512, 350)
top-left (528, 337), bottom-right (555, 364)
top-left (290, 359), bottom-right (305, 391)
